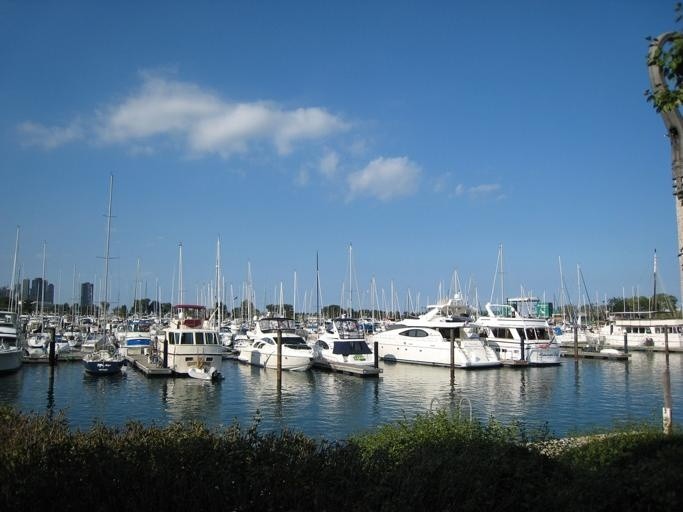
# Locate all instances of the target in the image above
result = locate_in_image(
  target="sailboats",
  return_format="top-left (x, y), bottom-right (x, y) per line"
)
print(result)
top-left (0, 173), bottom-right (681, 384)
